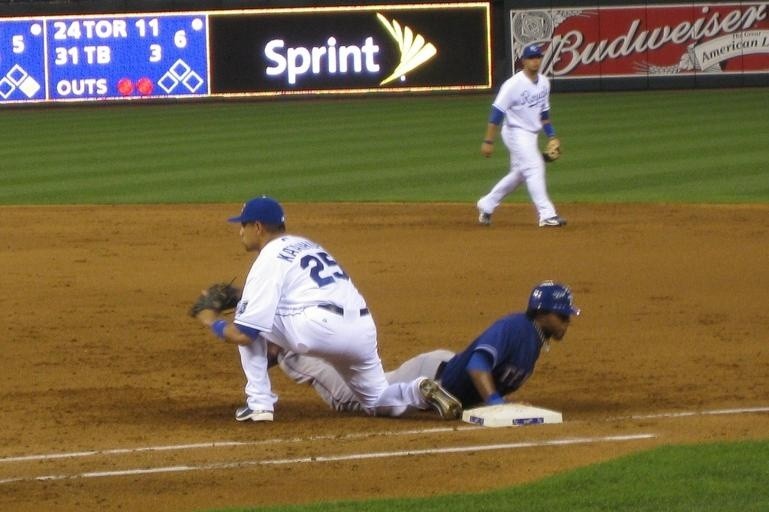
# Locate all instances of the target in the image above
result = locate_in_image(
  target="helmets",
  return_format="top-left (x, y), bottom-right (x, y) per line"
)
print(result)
top-left (528, 280), bottom-right (580, 316)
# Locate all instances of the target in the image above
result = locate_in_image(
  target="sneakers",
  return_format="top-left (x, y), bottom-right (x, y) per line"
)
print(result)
top-left (477, 201), bottom-right (491, 224)
top-left (415, 377), bottom-right (462, 420)
top-left (538, 215), bottom-right (566, 227)
top-left (236, 407), bottom-right (274, 421)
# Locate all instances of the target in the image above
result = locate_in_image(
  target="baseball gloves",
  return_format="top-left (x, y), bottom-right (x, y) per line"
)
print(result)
top-left (189, 276), bottom-right (240, 318)
top-left (542, 139), bottom-right (562, 161)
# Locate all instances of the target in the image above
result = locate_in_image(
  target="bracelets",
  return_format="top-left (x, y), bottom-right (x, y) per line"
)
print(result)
top-left (485, 392), bottom-right (506, 404)
top-left (212, 319), bottom-right (229, 339)
top-left (483, 140), bottom-right (494, 145)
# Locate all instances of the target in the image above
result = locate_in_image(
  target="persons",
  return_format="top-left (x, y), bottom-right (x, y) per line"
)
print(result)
top-left (266, 278), bottom-right (580, 412)
top-left (186, 195), bottom-right (463, 423)
top-left (476, 45), bottom-right (568, 227)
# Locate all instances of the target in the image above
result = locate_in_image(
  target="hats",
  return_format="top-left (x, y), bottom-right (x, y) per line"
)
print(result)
top-left (227, 198), bottom-right (285, 221)
top-left (524, 46), bottom-right (544, 58)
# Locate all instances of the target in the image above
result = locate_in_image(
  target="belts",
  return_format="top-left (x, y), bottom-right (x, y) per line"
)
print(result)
top-left (320, 304), bottom-right (368, 315)
top-left (434, 361), bottom-right (445, 383)
